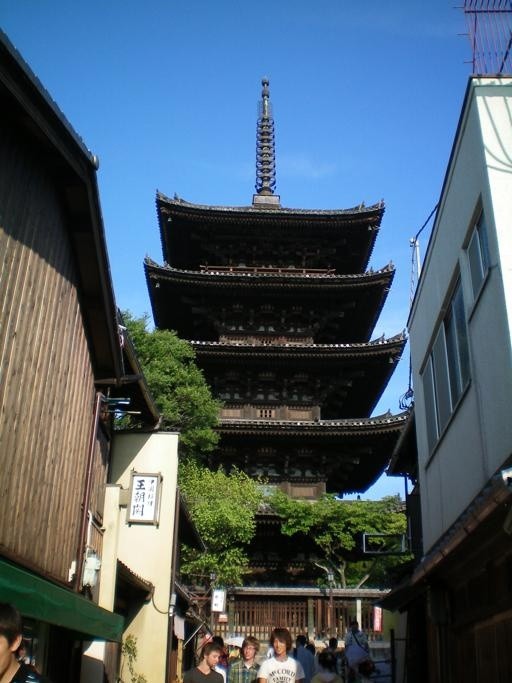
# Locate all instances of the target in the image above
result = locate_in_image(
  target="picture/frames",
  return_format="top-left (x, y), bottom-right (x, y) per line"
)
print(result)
top-left (125, 471), bottom-right (162, 526)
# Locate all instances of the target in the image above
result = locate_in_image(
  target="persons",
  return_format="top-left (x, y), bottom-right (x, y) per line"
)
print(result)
top-left (182, 618), bottom-right (372, 682)
top-left (0, 599), bottom-right (47, 682)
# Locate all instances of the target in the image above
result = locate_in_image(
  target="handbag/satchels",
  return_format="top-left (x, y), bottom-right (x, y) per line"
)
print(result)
top-left (345, 645), bottom-right (368, 668)
